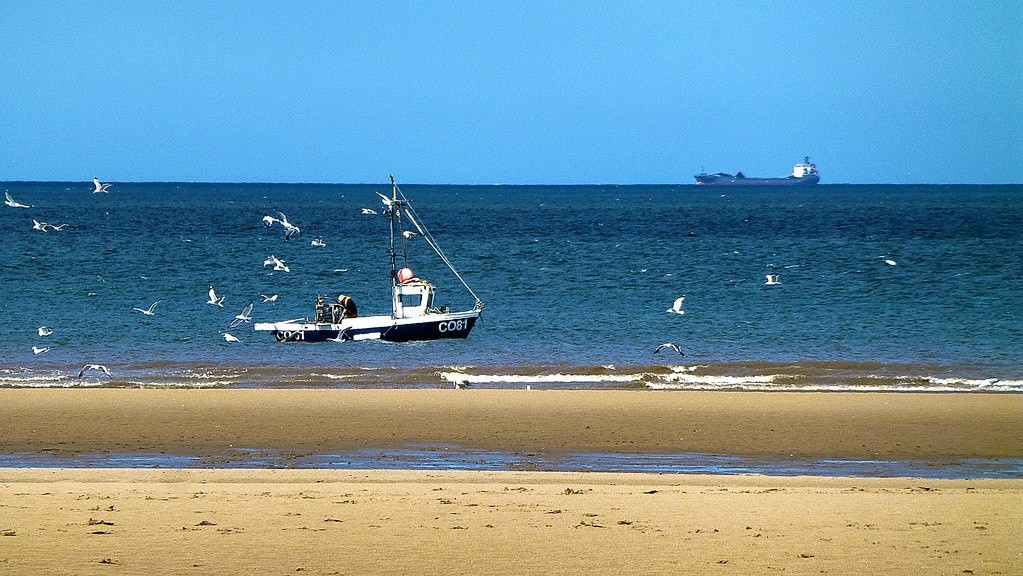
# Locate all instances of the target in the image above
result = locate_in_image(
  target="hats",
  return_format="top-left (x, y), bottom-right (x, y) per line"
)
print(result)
top-left (338, 295), bottom-right (346, 302)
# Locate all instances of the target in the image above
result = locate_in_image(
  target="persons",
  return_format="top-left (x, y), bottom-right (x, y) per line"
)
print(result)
top-left (338, 295), bottom-right (357, 318)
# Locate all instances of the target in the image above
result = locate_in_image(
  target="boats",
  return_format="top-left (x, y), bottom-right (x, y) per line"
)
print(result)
top-left (253, 173), bottom-right (484, 342)
top-left (693, 154), bottom-right (820, 185)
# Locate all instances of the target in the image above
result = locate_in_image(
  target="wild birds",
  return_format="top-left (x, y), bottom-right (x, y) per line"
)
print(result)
top-left (764, 275), bottom-right (782, 286)
top-left (93, 177), bottom-right (114, 194)
top-left (360, 208), bottom-right (377, 215)
top-left (37, 327), bottom-right (53, 336)
top-left (4, 190), bottom-right (30, 208)
top-left (375, 191), bottom-right (393, 207)
top-left (666, 297), bottom-right (686, 314)
top-left (223, 334), bottom-right (240, 343)
top-left (652, 343), bottom-right (687, 357)
top-left (78, 364), bottom-right (113, 379)
top-left (227, 303), bottom-right (253, 328)
top-left (134, 300), bottom-right (161, 316)
top-left (32, 219), bottom-right (69, 233)
top-left (403, 231), bottom-right (417, 240)
top-left (31, 345), bottom-right (50, 355)
top-left (258, 208), bottom-right (326, 302)
top-left (206, 288), bottom-right (225, 309)
top-left (326, 326), bottom-right (352, 344)
